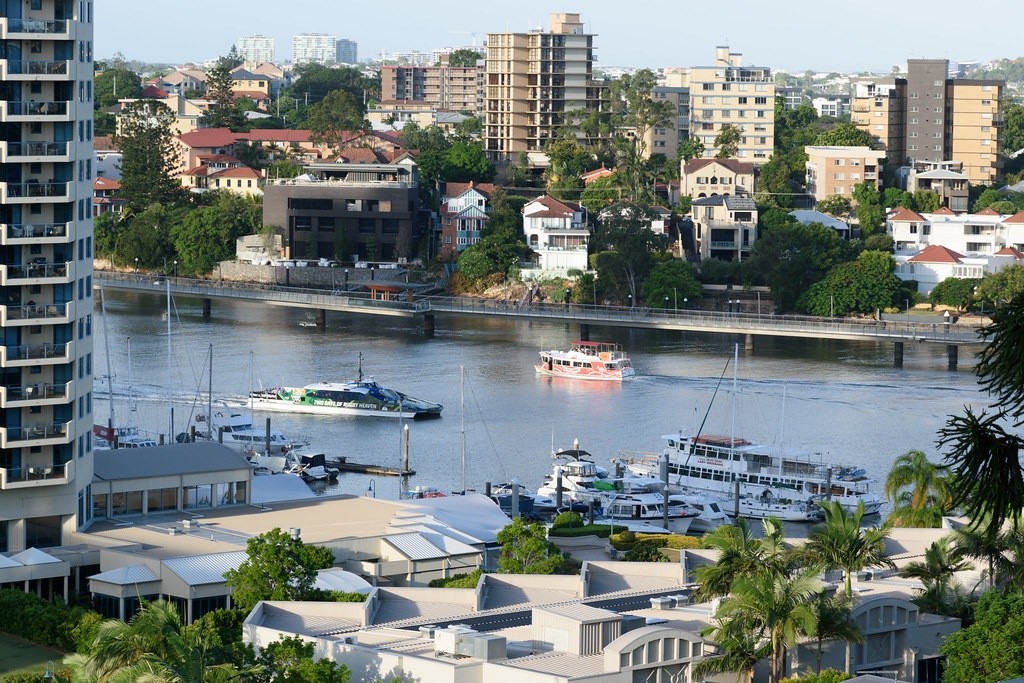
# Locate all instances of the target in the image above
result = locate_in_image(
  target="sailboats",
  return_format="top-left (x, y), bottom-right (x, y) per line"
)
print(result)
top-left (93, 279), bottom-right (329, 482)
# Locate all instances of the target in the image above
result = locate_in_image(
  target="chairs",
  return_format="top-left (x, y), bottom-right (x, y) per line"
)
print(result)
top-left (24, 225), bottom-right (35, 237)
top-left (12, 225), bottom-right (23, 238)
top-left (29, 468), bottom-right (51, 479)
top-left (60, 423), bottom-right (67, 437)
top-left (29, 143), bottom-right (36, 155)
top-left (355, 262), bottom-right (398, 270)
top-left (44, 383), bottom-right (55, 396)
top-left (36, 143), bottom-right (44, 155)
top-left (39, 343), bottom-right (51, 358)
top-left (47, 143), bottom-right (59, 156)
top-left (33, 428), bottom-right (44, 439)
top-left (272, 257), bottom-right (331, 267)
top-left (26, 62), bottom-right (61, 74)
top-left (28, 178), bottom-right (57, 196)
top-left (17, 345), bottom-right (31, 359)
top-left (21, 20), bottom-right (56, 34)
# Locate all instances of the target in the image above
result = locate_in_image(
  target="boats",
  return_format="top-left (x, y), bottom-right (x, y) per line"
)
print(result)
top-left (246, 351), bottom-right (443, 421)
top-left (533, 340), bottom-right (636, 382)
top-left (410, 342), bottom-right (887, 540)
